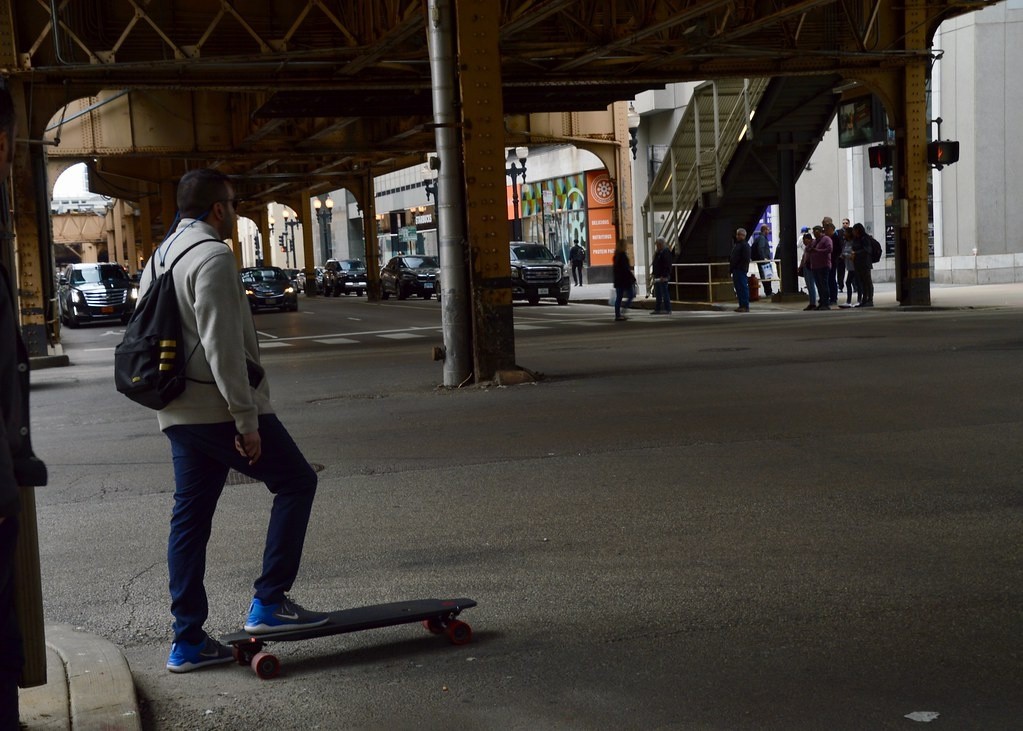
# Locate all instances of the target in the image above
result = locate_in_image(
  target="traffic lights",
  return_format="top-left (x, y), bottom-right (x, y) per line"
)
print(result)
top-left (254, 236), bottom-right (260, 249)
top-left (867, 145), bottom-right (893, 169)
top-left (290, 240), bottom-right (293, 251)
top-left (927, 140), bottom-right (961, 166)
top-left (282, 246), bottom-right (287, 252)
top-left (278, 236), bottom-right (284, 246)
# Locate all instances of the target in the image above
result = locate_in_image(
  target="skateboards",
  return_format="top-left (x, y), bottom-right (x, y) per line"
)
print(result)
top-left (219, 599), bottom-right (477, 680)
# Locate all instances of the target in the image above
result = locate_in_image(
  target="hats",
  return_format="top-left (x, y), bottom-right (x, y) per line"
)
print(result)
top-left (801, 227), bottom-right (811, 232)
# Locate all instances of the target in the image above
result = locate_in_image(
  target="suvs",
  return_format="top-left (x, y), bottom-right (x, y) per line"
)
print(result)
top-left (510, 243), bottom-right (571, 307)
top-left (57, 261), bottom-right (140, 328)
top-left (323, 258), bottom-right (367, 297)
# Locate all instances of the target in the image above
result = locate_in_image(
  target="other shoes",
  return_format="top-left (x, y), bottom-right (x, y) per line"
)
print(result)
top-left (579, 284), bottom-right (583, 286)
top-left (574, 283), bottom-right (578, 286)
top-left (853, 303), bottom-right (861, 308)
top-left (814, 303), bottom-right (830, 310)
top-left (649, 310), bottom-right (672, 314)
top-left (855, 302), bottom-right (873, 308)
top-left (615, 316), bottom-right (627, 321)
top-left (734, 306), bottom-right (750, 312)
top-left (838, 303), bottom-right (851, 308)
top-left (803, 304), bottom-right (816, 311)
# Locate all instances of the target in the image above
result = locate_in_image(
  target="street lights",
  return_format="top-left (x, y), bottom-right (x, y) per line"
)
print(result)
top-left (505, 147), bottom-right (530, 241)
top-left (421, 166), bottom-right (440, 268)
top-left (313, 196), bottom-right (334, 262)
top-left (282, 209), bottom-right (301, 268)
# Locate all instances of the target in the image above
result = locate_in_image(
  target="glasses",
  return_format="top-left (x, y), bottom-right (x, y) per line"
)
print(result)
top-left (736, 233), bottom-right (742, 235)
top-left (215, 199), bottom-right (239, 211)
top-left (843, 223), bottom-right (849, 224)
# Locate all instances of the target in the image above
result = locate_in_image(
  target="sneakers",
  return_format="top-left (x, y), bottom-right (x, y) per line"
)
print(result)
top-left (167, 631), bottom-right (234, 673)
top-left (244, 594), bottom-right (329, 633)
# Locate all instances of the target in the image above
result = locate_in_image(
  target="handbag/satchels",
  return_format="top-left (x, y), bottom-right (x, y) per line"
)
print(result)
top-left (608, 289), bottom-right (617, 305)
top-left (762, 263), bottom-right (774, 279)
top-left (798, 266), bottom-right (804, 277)
top-left (804, 258), bottom-right (811, 270)
top-left (633, 283), bottom-right (639, 295)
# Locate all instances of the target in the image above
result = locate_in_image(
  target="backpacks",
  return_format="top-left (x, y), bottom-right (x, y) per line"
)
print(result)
top-left (114, 241), bottom-right (231, 410)
top-left (861, 235), bottom-right (882, 263)
top-left (577, 248), bottom-right (586, 261)
top-left (750, 237), bottom-right (765, 260)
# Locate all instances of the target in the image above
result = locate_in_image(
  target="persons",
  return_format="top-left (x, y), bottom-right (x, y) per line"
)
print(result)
top-left (754, 224), bottom-right (775, 298)
top-left (729, 228), bottom-right (751, 312)
top-left (775, 232), bottom-right (781, 279)
top-left (135, 168), bottom-right (330, 673)
top-left (797, 217), bottom-right (873, 310)
top-left (569, 239), bottom-right (586, 287)
top-left (613, 239), bottom-right (636, 321)
top-left (650, 236), bottom-right (672, 315)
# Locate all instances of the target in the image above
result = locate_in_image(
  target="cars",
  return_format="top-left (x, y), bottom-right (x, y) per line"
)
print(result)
top-left (297, 266), bottom-right (323, 295)
top-left (283, 268), bottom-right (301, 284)
top-left (380, 255), bottom-right (440, 301)
top-left (239, 267), bottom-right (298, 313)
